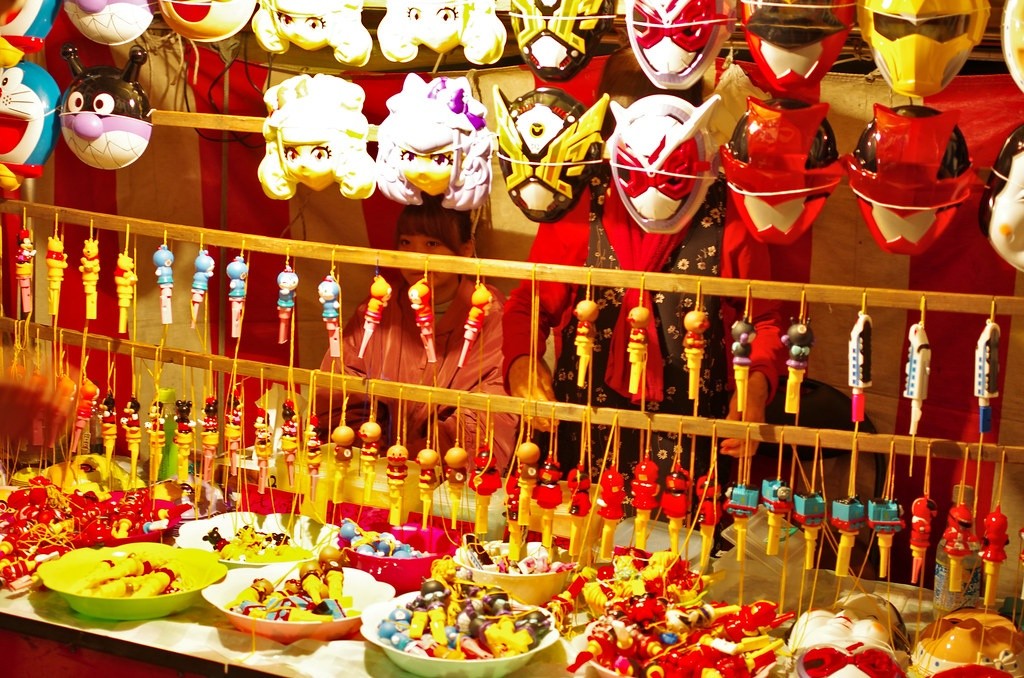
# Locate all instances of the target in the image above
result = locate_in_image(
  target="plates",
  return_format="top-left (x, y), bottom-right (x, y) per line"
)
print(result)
top-left (361, 592), bottom-right (559, 678)
top-left (37, 542), bottom-right (228, 620)
top-left (583, 620), bottom-right (776, 678)
top-left (176, 512), bottom-right (340, 570)
top-left (202, 563), bottom-right (396, 644)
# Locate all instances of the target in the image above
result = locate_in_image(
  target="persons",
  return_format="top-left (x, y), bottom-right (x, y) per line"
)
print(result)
top-left (500, 57), bottom-right (787, 530)
top-left (302, 192), bottom-right (521, 473)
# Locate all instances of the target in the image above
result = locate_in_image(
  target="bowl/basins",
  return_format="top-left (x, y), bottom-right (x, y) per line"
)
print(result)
top-left (454, 542), bottom-right (569, 605)
top-left (337, 524), bottom-right (458, 597)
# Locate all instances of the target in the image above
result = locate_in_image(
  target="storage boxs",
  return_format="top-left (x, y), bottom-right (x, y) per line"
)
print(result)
top-left (217, 424), bottom-right (715, 575)
